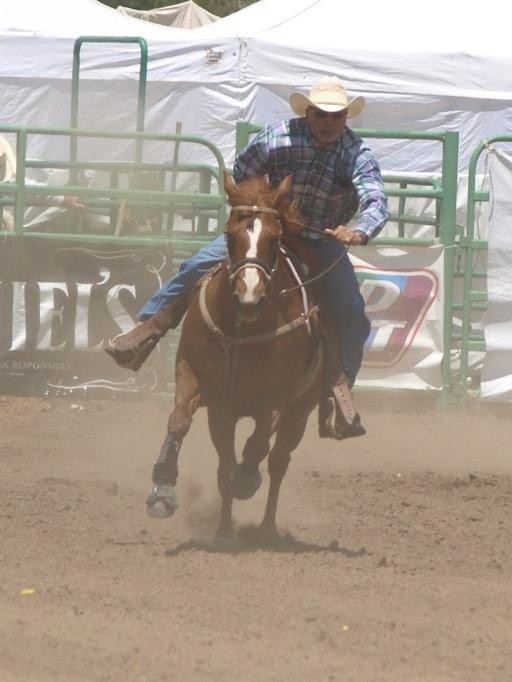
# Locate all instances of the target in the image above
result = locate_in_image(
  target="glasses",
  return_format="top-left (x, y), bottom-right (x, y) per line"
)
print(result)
top-left (313, 109), bottom-right (348, 118)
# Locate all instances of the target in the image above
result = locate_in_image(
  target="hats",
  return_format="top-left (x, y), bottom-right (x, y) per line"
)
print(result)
top-left (290, 76), bottom-right (366, 119)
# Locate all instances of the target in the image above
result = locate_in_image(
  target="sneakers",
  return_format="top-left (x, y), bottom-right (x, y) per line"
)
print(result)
top-left (318, 415), bottom-right (366, 438)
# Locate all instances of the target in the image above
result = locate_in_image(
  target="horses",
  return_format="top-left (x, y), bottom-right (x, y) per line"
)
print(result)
top-left (145, 170), bottom-right (328, 539)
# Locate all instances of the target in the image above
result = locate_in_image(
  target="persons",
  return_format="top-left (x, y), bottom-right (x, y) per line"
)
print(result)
top-left (9, 159), bottom-right (86, 209)
top-left (106, 82), bottom-right (390, 441)
